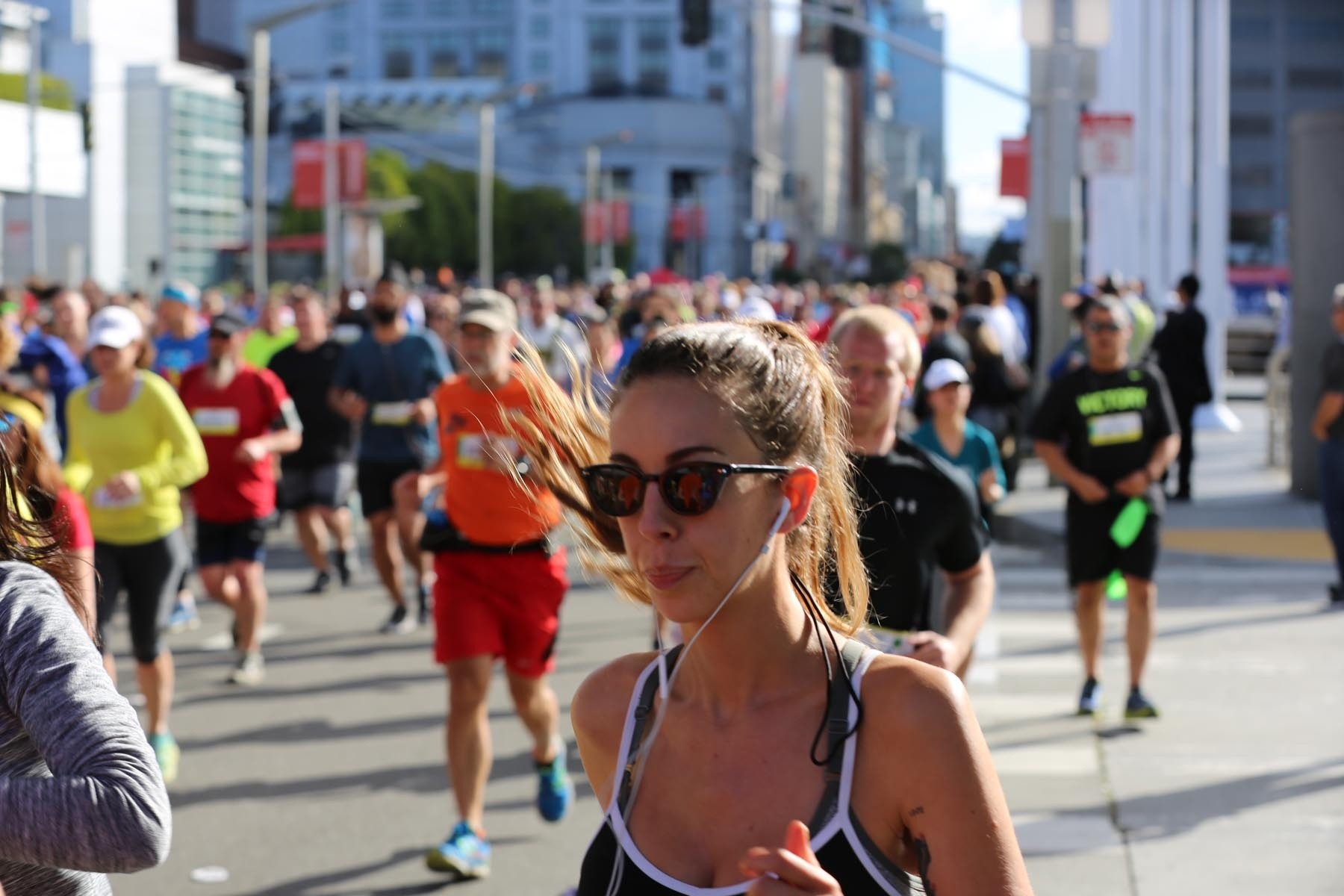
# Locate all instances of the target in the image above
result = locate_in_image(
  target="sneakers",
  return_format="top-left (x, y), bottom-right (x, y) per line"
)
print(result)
top-left (1125, 694), bottom-right (1158, 719)
top-left (428, 821), bottom-right (492, 881)
top-left (532, 733), bottom-right (572, 823)
top-left (165, 598), bottom-right (203, 632)
top-left (229, 655), bottom-right (263, 687)
top-left (148, 731), bottom-right (181, 785)
top-left (1079, 677), bottom-right (1102, 714)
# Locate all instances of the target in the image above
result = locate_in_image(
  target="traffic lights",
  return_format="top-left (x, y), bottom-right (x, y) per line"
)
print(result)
top-left (678, 0), bottom-right (712, 45)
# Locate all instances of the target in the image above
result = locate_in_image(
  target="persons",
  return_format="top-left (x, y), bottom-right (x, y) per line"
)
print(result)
top-left (1312, 281), bottom-right (1344, 605)
top-left (0, 267), bottom-right (1210, 782)
top-left (571, 314), bottom-right (1036, 896)
top-left (393, 288), bottom-right (575, 879)
top-left (0, 439), bottom-right (173, 896)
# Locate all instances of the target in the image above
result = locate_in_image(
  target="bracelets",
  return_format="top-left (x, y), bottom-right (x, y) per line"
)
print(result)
top-left (1140, 468), bottom-right (1153, 485)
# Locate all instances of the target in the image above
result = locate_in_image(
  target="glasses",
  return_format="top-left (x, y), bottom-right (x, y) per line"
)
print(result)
top-left (1084, 317), bottom-right (1130, 333)
top-left (580, 461), bottom-right (797, 518)
top-left (207, 330), bottom-right (230, 340)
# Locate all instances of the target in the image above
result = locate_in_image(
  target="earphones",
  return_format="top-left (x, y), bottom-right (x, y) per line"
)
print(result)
top-left (766, 495), bottom-right (791, 537)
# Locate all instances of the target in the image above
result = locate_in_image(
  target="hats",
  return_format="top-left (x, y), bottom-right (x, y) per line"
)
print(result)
top-left (208, 309), bottom-right (250, 331)
top-left (456, 289), bottom-right (519, 331)
top-left (924, 358), bottom-right (970, 389)
top-left (85, 305), bottom-right (145, 357)
